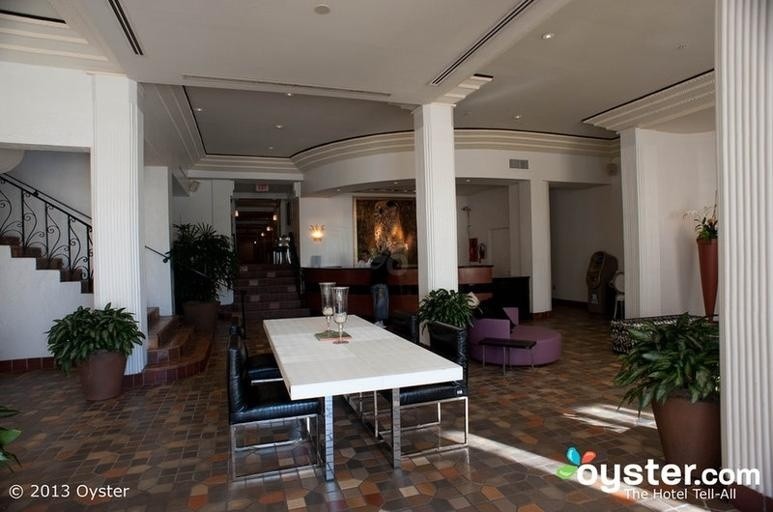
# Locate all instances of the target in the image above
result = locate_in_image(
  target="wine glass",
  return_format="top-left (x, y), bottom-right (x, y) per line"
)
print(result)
top-left (318, 282), bottom-right (336, 332)
top-left (330, 287), bottom-right (350, 344)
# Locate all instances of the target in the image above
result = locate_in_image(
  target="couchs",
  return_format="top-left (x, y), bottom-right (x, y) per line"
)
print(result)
top-left (470, 308), bottom-right (562, 366)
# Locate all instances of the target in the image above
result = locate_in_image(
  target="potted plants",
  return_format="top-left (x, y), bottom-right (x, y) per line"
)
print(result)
top-left (693, 218), bottom-right (718, 322)
top-left (43, 303), bottom-right (145, 402)
top-left (611, 312), bottom-right (718, 484)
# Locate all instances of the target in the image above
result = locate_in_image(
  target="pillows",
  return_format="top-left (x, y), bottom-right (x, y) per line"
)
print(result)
top-left (473, 297), bottom-right (516, 330)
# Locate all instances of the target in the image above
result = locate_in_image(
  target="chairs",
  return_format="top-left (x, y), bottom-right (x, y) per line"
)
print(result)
top-left (373, 322), bottom-right (471, 469)
top-left (227, 335), bottom-right (321, 482)
top-left (230, 315), bottom-right (289, 383)
top-left (346, 310), bottom-right (419, 420)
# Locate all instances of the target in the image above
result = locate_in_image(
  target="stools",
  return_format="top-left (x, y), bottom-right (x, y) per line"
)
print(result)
top-left (478, 338), bottom-right (537, 375)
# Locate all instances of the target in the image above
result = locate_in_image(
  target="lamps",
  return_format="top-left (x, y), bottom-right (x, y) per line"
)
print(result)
top-left (309, 225), bottom-right (326, 242)
